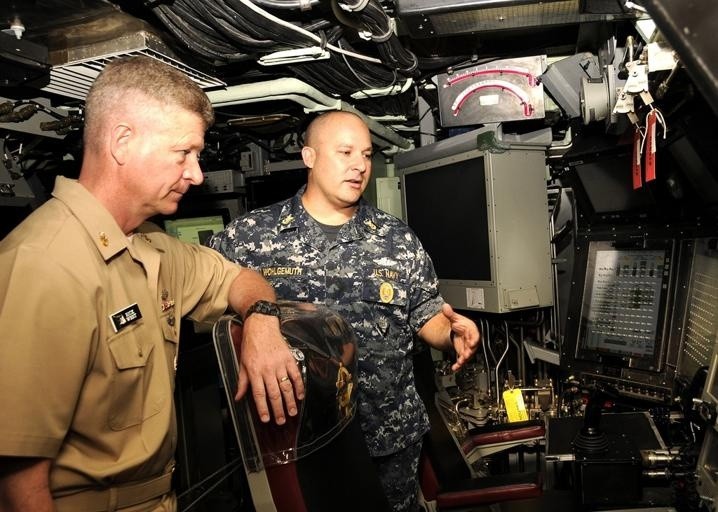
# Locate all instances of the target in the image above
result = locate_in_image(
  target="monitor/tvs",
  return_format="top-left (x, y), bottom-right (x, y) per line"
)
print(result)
top-left (396, 146), bottom-right (554, 314)
top-left (157, 208), bottom-right (230, 245)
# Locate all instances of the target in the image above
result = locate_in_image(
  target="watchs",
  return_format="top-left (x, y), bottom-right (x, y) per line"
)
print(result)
top-left (244, 298), bottom-right (285, 320)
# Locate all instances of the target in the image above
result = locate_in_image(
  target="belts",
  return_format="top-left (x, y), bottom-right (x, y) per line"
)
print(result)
top-left (54, 465), bottom-right (177, 512)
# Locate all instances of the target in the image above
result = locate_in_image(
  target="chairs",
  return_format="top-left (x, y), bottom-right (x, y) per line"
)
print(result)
top-left (411, 328), bottom-right (546, 510)
top-left (211, 307), bottom-right (395, 512)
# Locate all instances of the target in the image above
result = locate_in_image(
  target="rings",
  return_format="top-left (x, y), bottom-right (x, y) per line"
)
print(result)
top-left (277, 376), bottom-right (292, 383)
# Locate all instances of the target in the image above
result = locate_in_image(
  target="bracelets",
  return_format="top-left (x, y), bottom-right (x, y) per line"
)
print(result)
top-left (448, 322), bottom-right (457, 350)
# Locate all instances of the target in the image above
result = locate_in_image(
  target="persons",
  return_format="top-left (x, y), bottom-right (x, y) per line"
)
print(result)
top-left (204, 111), bottom-right (482, 512)
top-left (3, 57), bottom-right (304, 512)
